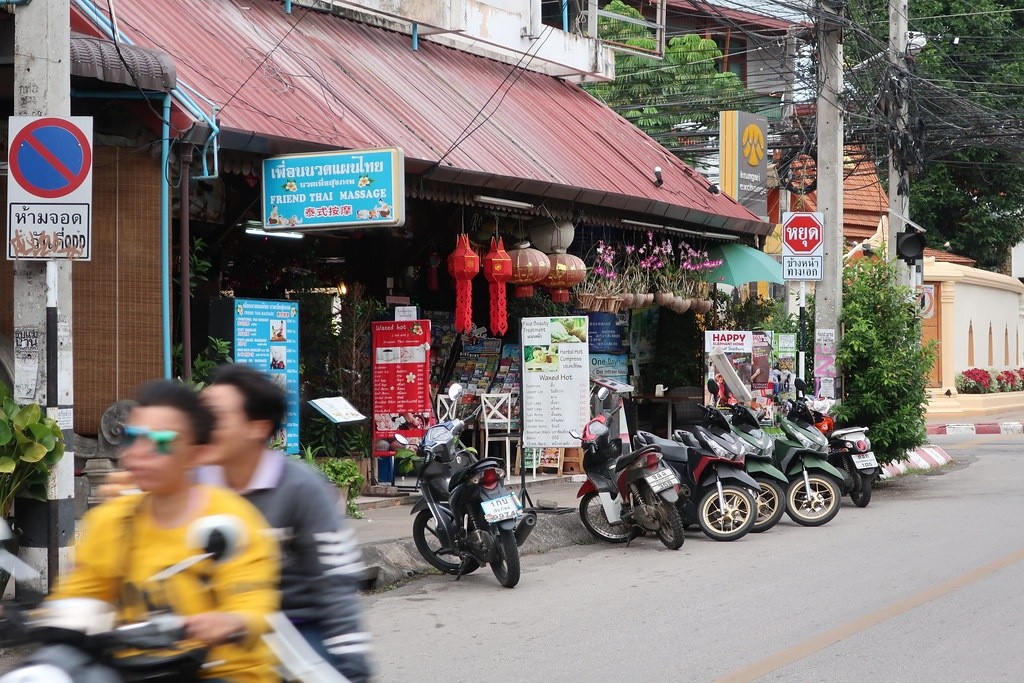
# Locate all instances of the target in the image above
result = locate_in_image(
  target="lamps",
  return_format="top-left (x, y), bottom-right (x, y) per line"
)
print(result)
top-left (244, 224), bottom-right (304, 239)
top-left (474, 195), bottom-right (533, 210)
top-left (654, 166), bottom-right (664, 187)
top-left (620, 219), bottom-right (741, 240)
top-left (708, 185), bottom-right (721, 196)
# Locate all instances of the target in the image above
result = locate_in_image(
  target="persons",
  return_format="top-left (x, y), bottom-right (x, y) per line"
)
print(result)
top-left (773, 365), bottom-right (793, 395)
top-left (20, 373), bottom-right (283, 683)
top-left (182, 364), bottom-right (374, 683)
top-left (708, 373), bottom-right (726, 408)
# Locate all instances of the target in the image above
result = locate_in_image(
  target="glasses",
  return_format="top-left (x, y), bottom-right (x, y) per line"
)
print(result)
top-left (119, 425), bottom-right (177, 455)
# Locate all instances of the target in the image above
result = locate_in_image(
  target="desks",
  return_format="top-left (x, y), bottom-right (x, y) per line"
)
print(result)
top-left (631, 394), bottom-right (702, 440)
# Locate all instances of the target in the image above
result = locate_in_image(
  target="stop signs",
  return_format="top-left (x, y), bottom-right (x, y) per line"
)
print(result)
top-left (783, 213), bottom-right (823, 255)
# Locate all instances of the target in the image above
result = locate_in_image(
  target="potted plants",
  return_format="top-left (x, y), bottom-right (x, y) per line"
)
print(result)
top-left (0, 380), bottom-right (65, 602)
top-left (341, 426), bottom-right (372, 495)
top-left (320, 457), bottom-right (365, 519)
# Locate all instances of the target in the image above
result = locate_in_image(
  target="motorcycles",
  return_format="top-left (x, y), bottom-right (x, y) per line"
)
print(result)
top-left (0, 513), bottom-right (249, 683)
top-left (632, 377), bottom-right (882, 543)
top-left (569, 385), bottom-right (690, 551)
top-left (394, 384), bottom-right (538, 589)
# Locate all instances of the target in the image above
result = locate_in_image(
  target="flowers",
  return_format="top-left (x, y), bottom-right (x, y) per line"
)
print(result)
top-left (956, 368), bottom-right (1024, 394)
top-left (570, 230), bottom-right (724, 302)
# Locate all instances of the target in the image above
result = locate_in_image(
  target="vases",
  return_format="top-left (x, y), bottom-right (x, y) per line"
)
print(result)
top-left (971, 387), bottom-right (1003, 393)
top-left (570, 292), bottom-right (710, 314)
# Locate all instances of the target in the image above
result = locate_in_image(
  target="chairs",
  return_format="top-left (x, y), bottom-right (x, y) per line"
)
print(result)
top-left (671, 386), bottom-right (704, 430)
top-left (481, 394), bottom-right (522, 482)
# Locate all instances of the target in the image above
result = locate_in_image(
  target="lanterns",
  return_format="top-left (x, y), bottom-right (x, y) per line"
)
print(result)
top-left (507, 240), bottom-right (549, 297)
top-left (485, 235), bottom-right (512, 334)
top-left (446, 231), bottom-right (480, 334)
top-left (541, 248), bottom-right (586, 302)
